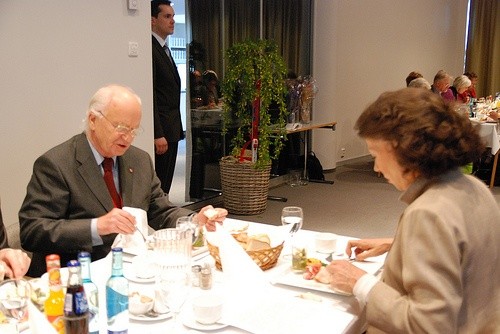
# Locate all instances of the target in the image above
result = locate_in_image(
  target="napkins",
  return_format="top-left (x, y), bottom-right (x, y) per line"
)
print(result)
top-left (111, 206), bottom-right (148, 256)
top-left (214, 220), bottom-right (272, 299)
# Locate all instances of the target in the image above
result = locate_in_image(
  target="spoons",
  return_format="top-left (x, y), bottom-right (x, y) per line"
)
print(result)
top-left (199, 316), bottom-right (254, 334)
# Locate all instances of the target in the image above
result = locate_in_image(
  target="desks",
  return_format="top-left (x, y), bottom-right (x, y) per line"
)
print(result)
top-left (34, 218), bottom-right (388, 333)
top-left (267, 120), bottom-right (337, 203)
top-left (467, 102), bottom-right (500, 189)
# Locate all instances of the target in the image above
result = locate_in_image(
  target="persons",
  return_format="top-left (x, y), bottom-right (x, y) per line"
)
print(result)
top-left (189, 69), bottom-right (220, 110)
top-left (406, 71), bottom-right (478, 101)
top-left (151, 0), bottom-right (185, 197)
top-left (0, 209), bottom-right (31, 283)
top-left (20, 84), bottom-right (228, 279)
top-left (325, 87), bottom-right (500, 334)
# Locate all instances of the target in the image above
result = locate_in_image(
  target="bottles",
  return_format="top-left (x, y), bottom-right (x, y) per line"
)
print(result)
top-left (76, 252), bottom-right (99, 334)
top-left (62, 260), bottom-right (91, 334)
top-left (44, 254), bottom-right (68, 334)
top-left (107, 249), bottom-right (128, 333)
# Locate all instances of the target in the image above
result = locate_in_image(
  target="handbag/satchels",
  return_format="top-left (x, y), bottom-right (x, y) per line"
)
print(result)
top-left (301, 151), bottom-right (326, 181)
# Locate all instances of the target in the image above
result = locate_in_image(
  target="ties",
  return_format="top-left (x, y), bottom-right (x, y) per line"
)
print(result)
top-left (100, 155), bottom-right (122, 209)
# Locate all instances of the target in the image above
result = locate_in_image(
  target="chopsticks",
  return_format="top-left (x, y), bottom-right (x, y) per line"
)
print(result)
top-left (193, 249), bottom-right (211, 261)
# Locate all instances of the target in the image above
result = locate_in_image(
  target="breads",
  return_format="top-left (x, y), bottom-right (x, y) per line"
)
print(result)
top-left (203, 209), bottom-right (219, 220)
top-left (231, 232), bottom-right (270, 252)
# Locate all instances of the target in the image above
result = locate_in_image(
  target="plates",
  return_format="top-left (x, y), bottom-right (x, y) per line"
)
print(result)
top-left (183, 306), bottom-right (230, 330)
top-left (123, 254), bottom-right (175, 321)
top-left (276, 256), bottom-right (354, 297)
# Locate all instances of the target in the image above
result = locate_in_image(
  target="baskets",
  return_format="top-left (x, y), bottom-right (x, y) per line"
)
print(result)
top-left (218, 156), bottom-right (272, 215)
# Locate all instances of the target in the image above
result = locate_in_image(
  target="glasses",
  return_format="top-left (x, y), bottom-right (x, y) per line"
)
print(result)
top-left (97, 110), bottom-right (142, 137)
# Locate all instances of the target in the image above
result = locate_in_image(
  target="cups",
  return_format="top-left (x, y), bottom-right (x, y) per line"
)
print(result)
top-left (193, 255), bottom-right (216, 288)
top-left (177, 216), bottom-right (198, 249)
top-left (0, 277), bottom-right (34, 330)
top-left (280, 207), bottom-right (304, 236)
top-left (154, 230), bottom-right (177, 252)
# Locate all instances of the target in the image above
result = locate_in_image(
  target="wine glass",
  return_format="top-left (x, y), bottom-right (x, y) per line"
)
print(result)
top-left (472, 105), bottom-right (477, 118)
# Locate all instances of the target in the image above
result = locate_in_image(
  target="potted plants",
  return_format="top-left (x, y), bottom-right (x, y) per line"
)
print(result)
top-left (218, 36), bottom-right (296, 218)
